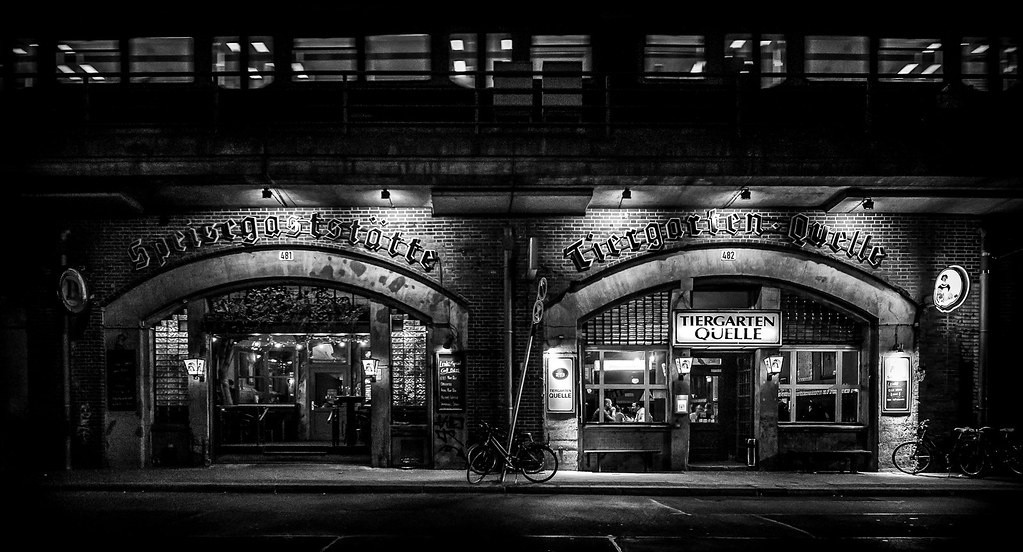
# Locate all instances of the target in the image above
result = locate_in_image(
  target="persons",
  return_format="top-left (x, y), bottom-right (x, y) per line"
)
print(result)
top-left (228, 378), bottom-right (279, 404)
top-left (688, 402), bottom-right (712, 422)
top-left (779, 389), bottom-right (829, 422)
top-left (593, 398), bottom-right (653, 423)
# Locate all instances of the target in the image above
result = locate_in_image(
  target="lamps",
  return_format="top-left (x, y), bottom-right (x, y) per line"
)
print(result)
top-left (381, 187), bottom-right (395, 209)
top-left (673, 352), bottom-right (692, 381)
top-left (726, 186), bottom-right (751, 207)
top-left (184, 355), bottom-right (206, 382)
top-left (262, 188), bottom-right (284, 208)
top-left (618, 188), bottom-right (631, 209)
top-left (762, 349), bottom-right (784, 381)
top-left (846, 191), bottom-right (874, 213)
top-left (441, 334), bottom-right (454, 349)
top-left (361, 355), bottom-right (381, 383)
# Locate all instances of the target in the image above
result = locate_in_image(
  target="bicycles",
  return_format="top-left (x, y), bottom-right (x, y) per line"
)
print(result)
top-left (891, 419), bottom-right (1023, 479)
top-left (466, 420), bottom-right (559, 485)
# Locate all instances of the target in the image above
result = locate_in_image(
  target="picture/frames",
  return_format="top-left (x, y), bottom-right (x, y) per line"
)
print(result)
top-left (880, 355), bottom-right (911, 415)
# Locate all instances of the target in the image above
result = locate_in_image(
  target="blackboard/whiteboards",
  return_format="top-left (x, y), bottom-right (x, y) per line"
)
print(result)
top-left (105, 350), bottom-right (137, 411)
top-left (437, 351), bottom-right (463, 413)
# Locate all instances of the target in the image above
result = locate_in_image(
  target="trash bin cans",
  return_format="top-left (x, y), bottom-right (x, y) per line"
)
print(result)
top-left (747, 438), bottom-right (757, 468)
